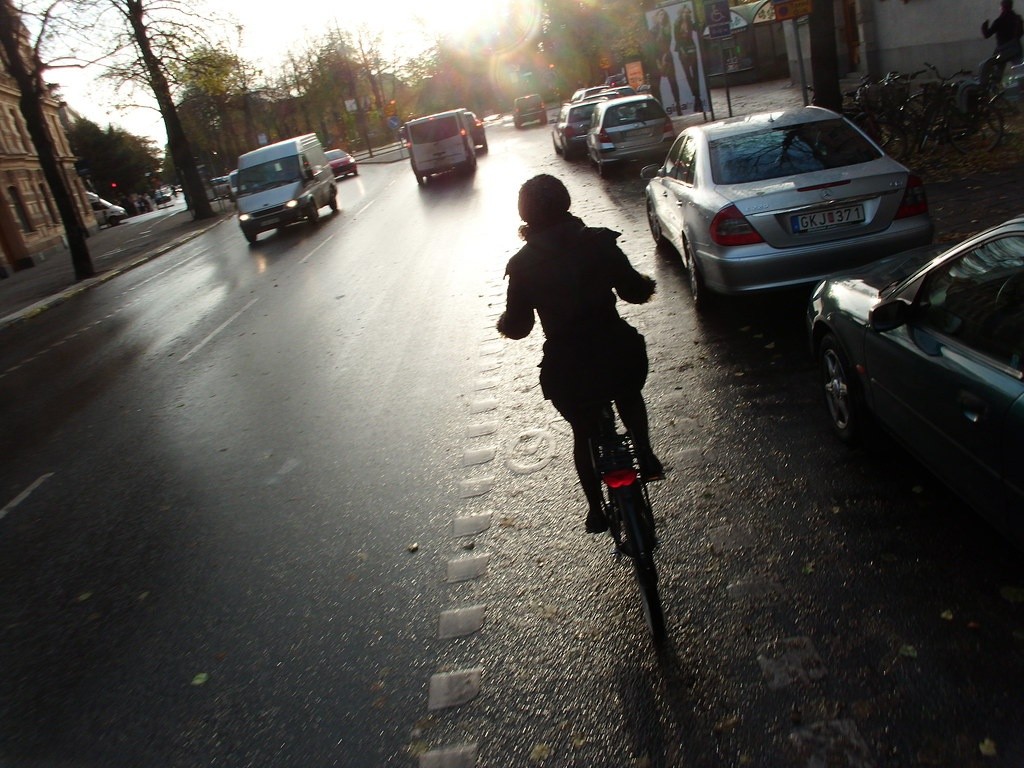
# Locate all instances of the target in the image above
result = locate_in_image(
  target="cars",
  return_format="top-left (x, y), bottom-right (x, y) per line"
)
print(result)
top-left (463, 111), bottom-right (488, 151)
top-left (586, 95), bottom-right (677, 178)
top-left (603, 73), bottom-right (629, 88)
top-left (799, 208), bottom-right (1024, 542)
top-left (322, 148), bottom-right (358, 179)
top-left (550, 97), bottom-right (610, 161)
top-left (637, 104), bottom-right (934, 312)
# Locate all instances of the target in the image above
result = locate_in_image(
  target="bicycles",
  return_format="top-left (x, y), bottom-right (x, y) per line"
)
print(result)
top-left (497, 274), bottom-right (671, 634)
top-left (807, 54), bottom-right (1024, 170)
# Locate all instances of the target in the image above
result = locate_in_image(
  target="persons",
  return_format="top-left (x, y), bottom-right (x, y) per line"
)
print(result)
top-left (493, 176), bottom-right (664, 532)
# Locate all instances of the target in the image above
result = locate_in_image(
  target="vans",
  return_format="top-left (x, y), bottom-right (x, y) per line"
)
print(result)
top-left (210, 176), bottom-right (231, 199)
top-left (88, 196), bottom-right (128, 228)
top-left (236, 132), bottom-right (339, 244)
top-left (513, 94), bottom-right (546, 128)
top-left (404, 106), bottom-right (477, 186)
top-left (227, 169), bottom-right (248, 203)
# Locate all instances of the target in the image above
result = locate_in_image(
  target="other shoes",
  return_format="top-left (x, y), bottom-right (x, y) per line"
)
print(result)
top-left (638, 454), bottom-right (663, 482)
top-left (584, 510), bottom-right (608, 534)
